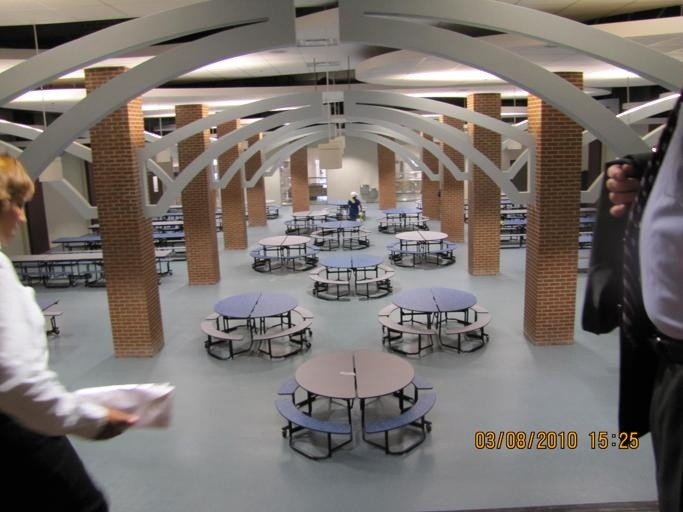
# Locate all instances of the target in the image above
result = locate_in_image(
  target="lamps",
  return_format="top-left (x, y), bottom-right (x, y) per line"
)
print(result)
top-left (33, 22), bottom-right (63, 184)
top-left (316, 70), bottom-right (346, 169)
top-left (507, 88), bottom-right (521, 150)
top-left (294, 0), bottom-right (340, 48)
top-left (156, 88), bottom-right (171, 162)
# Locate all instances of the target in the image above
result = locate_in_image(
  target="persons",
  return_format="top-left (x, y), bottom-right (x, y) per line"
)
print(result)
top-left (602, 82), bottom-right (683, 511)
top-left (346, 192), bottom-right (362, 220)
top-left (1, 151), bottom-right (143, 511)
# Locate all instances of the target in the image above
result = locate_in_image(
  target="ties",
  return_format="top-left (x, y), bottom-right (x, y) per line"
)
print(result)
top-left (620, 98), bottom-right (683, 324)
